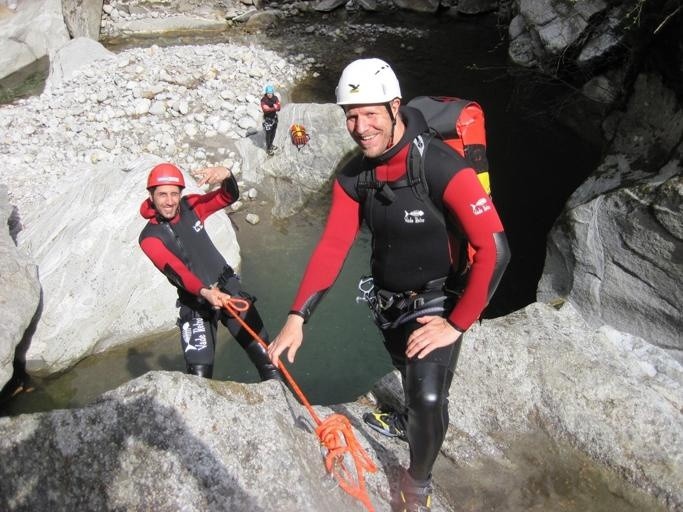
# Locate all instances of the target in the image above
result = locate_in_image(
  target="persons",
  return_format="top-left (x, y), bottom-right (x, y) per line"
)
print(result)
top-left (136, 162), bottom-right (281, 381)
top-left (260, 85), bottom-right (279, 157)
top-left (263, 58), bottom-right (513, 512)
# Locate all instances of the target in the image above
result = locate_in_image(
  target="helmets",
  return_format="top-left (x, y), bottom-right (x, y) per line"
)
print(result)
top-left (266, 85), bottom-right (274, 93)
top-left (334, 57), bottom-right (402, 107)
top-left (146, 162), bottom-right (186, 189)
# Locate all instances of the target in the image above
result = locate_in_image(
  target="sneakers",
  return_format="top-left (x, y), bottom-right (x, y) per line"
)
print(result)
top-left (266, 145), bottom-right (278, 156)
top-left (364, 407), bottom-right (413, 440)
top-left (396, 467), bottom-right (435, 512)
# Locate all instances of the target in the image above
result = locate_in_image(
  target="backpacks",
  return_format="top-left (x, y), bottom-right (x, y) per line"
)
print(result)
top-left (290, 123), bottom-right (310, 151)
top-left (405, 92), bottom-right (503, 283)
